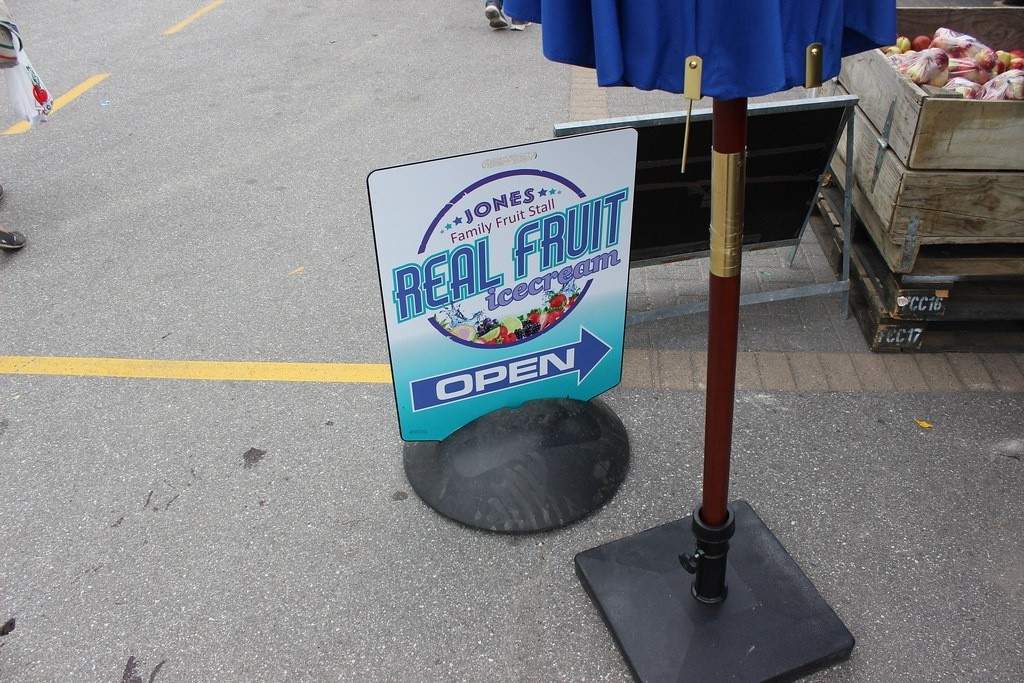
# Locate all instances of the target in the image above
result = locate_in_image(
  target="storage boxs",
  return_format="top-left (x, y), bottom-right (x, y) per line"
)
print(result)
top-left (816, 168), bottom-right (1024, 325)
top-left (808, 198), bottom-right (1024, 354)
top-left (819, 79), bottom-right (1024, 246)
top-left (837, 6), bottom-right (1024, 172)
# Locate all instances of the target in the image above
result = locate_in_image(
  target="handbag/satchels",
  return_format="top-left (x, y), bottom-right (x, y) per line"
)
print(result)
top-left (0, 0), bottom-right (23, 69)
top-left (4, 29), bottom-right (54, 129)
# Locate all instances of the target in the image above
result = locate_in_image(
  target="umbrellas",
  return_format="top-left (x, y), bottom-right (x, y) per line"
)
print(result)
top-left (503, 0), bottom-right (897, 604)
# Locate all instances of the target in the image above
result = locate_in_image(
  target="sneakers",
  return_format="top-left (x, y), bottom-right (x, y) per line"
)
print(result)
top-left (485, 2), bottom-right (508, 28)
top-left (510, 18), bottom-right (532, 31)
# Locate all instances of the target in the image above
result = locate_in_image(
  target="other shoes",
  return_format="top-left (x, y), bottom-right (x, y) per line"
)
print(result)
top-left (0, 230), bottom-right (26, 249)
top-left (0, 183), bottom-right (4, 199)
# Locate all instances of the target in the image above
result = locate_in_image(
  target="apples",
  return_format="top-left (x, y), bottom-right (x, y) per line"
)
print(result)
top-left (881, 28), bottom-right (1024, 100)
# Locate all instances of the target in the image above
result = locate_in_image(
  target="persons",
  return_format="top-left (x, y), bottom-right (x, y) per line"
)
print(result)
top-left (0, 0), bottom-right (28, 250)
top-left (485, 0), bottom-right (531, 31)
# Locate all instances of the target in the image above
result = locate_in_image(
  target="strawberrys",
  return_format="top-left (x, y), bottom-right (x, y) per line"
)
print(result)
top-left (473, 291), bottom-right (579, 345)
